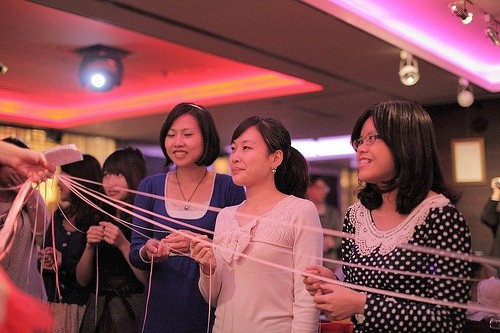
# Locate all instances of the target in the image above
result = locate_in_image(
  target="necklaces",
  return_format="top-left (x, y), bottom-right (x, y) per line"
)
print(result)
top-left (175, 169), bottom-right (208, 210)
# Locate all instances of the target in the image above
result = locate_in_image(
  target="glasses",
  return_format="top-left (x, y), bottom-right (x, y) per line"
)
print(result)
top-left (351, 133), bottom-right (384, 151)
top-left (311, 184), bottom-right (330, 193)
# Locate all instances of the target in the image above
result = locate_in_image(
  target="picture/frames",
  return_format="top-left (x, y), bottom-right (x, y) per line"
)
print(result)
top-left (450, 136), bottom-right (491, 187)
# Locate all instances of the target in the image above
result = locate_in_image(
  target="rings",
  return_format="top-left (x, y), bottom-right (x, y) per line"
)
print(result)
top-left (190, 243), bottom-right (194, 249)
top-left (103, 226), bottom-right (107, 230)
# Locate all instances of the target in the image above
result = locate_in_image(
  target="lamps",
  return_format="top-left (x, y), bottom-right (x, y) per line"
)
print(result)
top-left (448, 0), bottom-right (477, 28)
top-left (398, 50), bottom-right (421, 86)
top-left (483, 14), bottom-right (500, 45)
top-left (457, 78), bottom-right (474, 107)
top-left (73, 45), bottom-right (132, 92)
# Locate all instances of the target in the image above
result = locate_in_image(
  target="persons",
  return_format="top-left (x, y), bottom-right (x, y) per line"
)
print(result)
top-left (129, 102), bottom-right (246, 333)
top-left (74, 147), bottom-right (149, 333)
top-left (0, 140), bottom-right (56, 183)
top-left (189, 116), bottom-right (324, 333)
top-left (301, 100), bottom-right (475, 333)
top-left (480, 177), bottom-right (500, 260)
top-left (38, 154), bottom-right (115, 333)
top-left (0, 138), bottom-right (51, 301)
top-left (303, 175), bottom-right (342, 271)
